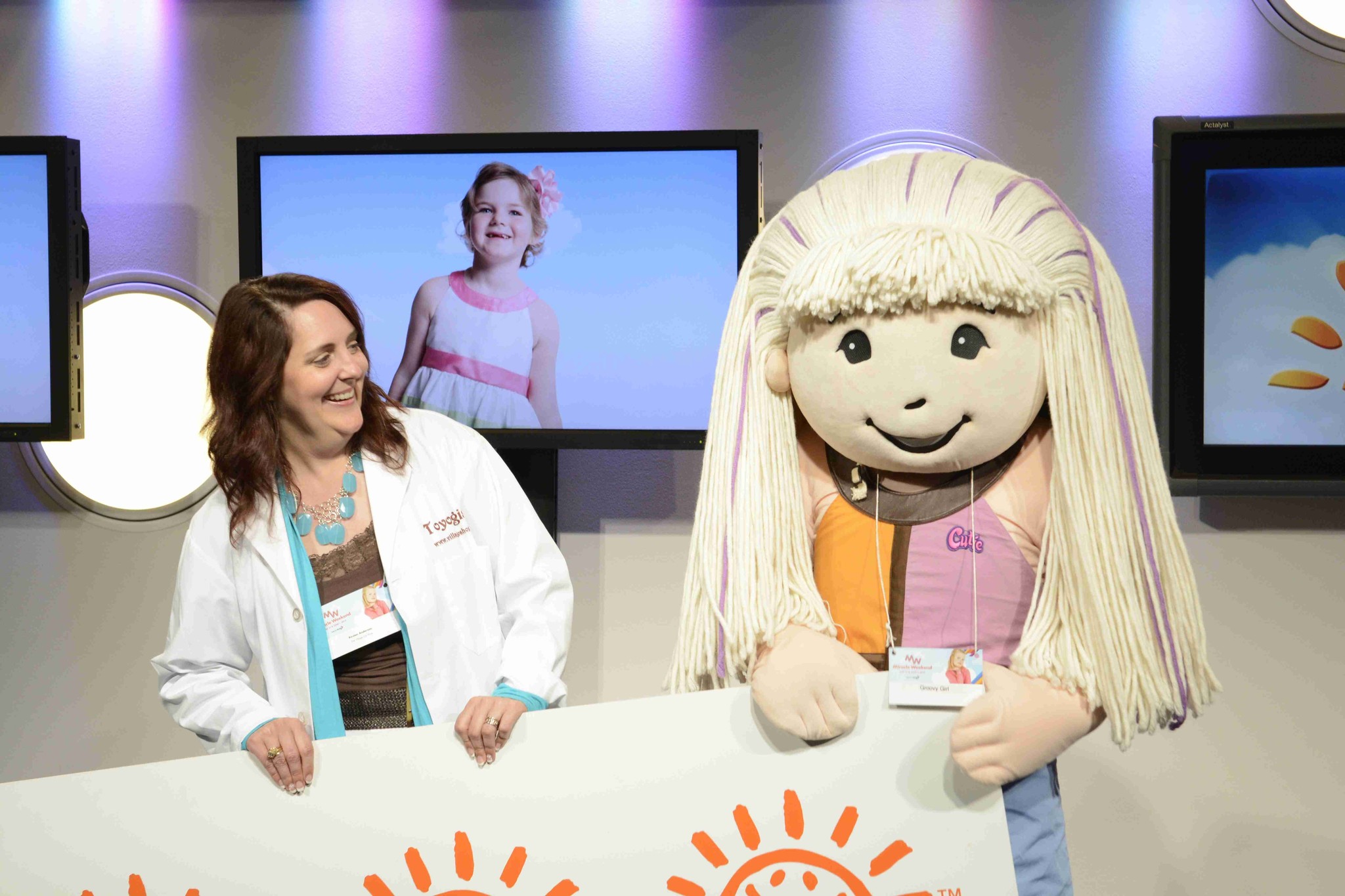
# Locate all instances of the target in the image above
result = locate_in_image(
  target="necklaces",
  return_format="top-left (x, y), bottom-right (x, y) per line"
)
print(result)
top-left (266, 446), bottom-right (370, 548)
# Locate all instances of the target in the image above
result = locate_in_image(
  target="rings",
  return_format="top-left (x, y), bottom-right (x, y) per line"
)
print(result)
top-left (266, 745), bottom-right (283, 759)
top-left (484, 717), bottom-right (500, 730)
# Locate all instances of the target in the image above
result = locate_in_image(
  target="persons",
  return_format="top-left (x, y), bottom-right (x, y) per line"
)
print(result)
top-left (378, 161), bottom-right (569, 428)
top-left (144, 272), bottom-right (578, 796)
top-left (622, 150), bottom-right (1228, 896)
top-left (944, 649), bottom-right (972, 685)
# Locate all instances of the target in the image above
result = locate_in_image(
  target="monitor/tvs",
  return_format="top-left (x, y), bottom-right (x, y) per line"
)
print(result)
top-left (1150, 110), bottom-right (1344, 500)
top-left (231, 128), bottom-right (765, 449)
top-left (0, 135), bottom-right (88, 448)
